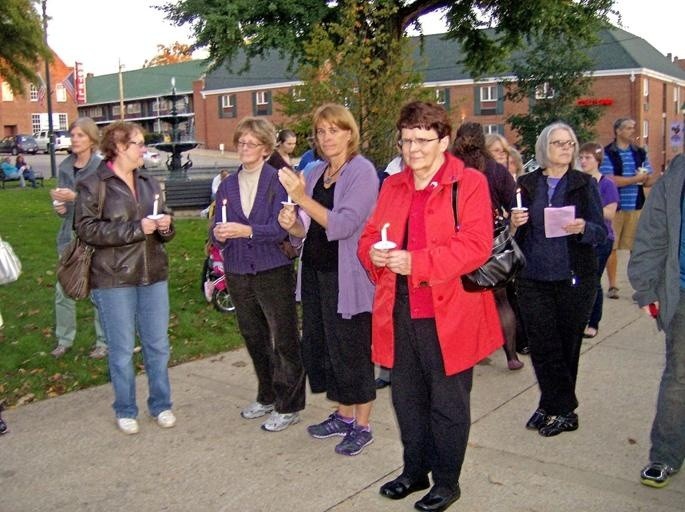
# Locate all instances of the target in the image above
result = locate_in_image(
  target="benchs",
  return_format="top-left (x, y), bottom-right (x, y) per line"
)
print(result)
top-left (0, 168), bottom-right (46, 190)
top-left (163, 178), bottom-right (213, 210)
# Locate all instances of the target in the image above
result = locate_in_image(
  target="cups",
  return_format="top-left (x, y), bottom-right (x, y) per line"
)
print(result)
top-left (374, 241), bottom-right (397, 257)
top-left (280, 201), bottom-right (297, 212)
top-left (147, 214), bottom-right (165, 230)
top-left (511, 207), bottom-right (528, 214)
top-left (636, 166), bottom-right (648, 186)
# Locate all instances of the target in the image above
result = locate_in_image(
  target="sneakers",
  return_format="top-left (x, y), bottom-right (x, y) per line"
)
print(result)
top-left (49, 346), bottom-right (72, 358)
top-left (156, 409), bottom-right (177, 429)
top-left (117, 416), bottom-right (139, 436)
top-left (639, 460), bottom-right (682, 489)
top-left (90, 346), bottom-right (107, 361)
top-left (260, 410), bottom-right (302, 433)
top-left (306, 410), bottom-right (356, 440)
top-left (335, 421), bottom-right (374, 456)
top-left (240, 401), bottom-right (276, 419)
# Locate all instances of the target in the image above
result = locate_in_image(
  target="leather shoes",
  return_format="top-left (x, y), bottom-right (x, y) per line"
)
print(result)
top-left (414, 485), bottom-right (461, 512)
top-left (526, 406), bottom-right (552, 430)
top-left (381, 470), bottom-right (431, 499)
top-left (540, 414), bottom-right (578, 437)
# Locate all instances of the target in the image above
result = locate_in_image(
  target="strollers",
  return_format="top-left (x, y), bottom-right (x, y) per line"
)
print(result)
top-left (202, 235), bottom-right (237, 311)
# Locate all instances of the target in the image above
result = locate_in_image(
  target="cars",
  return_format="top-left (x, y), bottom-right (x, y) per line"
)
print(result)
top-left (96, 145), bottom-right (163, 169)
top-left (0, 135), bottom-right (37, 155)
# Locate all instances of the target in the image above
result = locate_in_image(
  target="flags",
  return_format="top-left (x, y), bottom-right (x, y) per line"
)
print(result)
top-left (61, 70), bottom-right (77, 104)
top-left (36, 76), bottom-right (46, 108)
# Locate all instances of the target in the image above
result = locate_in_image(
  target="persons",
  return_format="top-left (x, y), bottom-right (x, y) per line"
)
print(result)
top-left (356, 98), bottom-right (505, 512)
top-left (15, 154), bottom-right (40, 190)
top-left (384, 131), bottom-right (407, 175)
top-left (48, 115), bottom-right (108, 361)
top-left (71, 119), bottom-right (178, 435)
top-left (453, 118), bottom-right (654, 438)
top-left (0, 156), bottom-right (27, 190)
top-left (207, 103), bottom-right (379, 455)
top-left (626, 152), bottom-right (685, 488)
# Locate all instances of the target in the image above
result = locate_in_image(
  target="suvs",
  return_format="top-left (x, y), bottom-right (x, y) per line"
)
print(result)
top-left (32, 130), bottom-right (70, 153)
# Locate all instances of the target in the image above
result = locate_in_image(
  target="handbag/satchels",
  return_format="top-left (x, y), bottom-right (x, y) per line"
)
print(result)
top-left (54, 178), bottom-right (108, 301)
top-left (278, 236), bottom-right (303, 260)
top-left (0, 235), bottom-right (24, 288)
top-left (452, 171), bottom-right (527, 293)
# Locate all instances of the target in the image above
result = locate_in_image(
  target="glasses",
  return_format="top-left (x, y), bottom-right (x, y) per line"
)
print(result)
top-left (126, 140), bottom-right (146, 149)
top-left (237, 140), bottom-right (267, 149)
top-left (549, 138), bottom-right (578, 150)
top-left (396, 135), bottom-right (441, 146)
